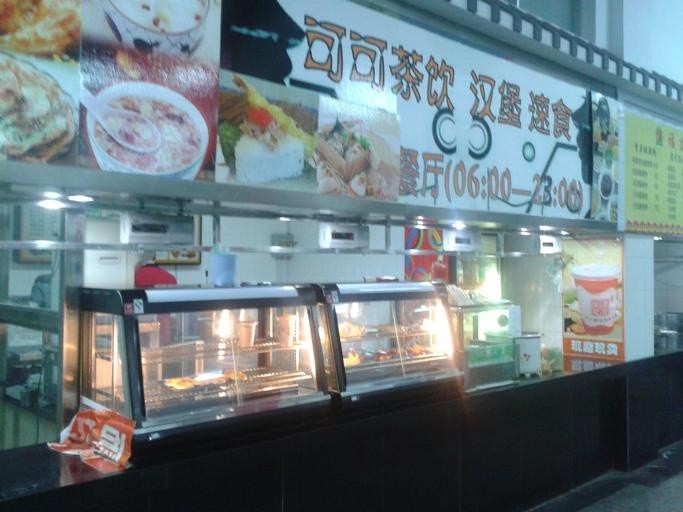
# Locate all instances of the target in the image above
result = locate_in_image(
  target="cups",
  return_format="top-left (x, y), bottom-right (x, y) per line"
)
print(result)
top-left (237, 320), bottom-right (259, 353)
top-left (274, 314), bottom-right (295, 348)
top-left (569, 263), bottom-right (620, 338)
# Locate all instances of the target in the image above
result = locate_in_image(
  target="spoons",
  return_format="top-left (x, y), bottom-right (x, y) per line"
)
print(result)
top-left (78, 87), bottom-right (168, 153)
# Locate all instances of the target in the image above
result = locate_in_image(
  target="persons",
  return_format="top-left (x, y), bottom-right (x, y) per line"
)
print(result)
top-left (134, 249), bottom-right (178, 349)
top-left (28, 273), bottom-right (52, 351)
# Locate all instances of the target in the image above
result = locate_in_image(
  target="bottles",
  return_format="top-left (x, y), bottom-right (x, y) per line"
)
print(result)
top-left (19, 384), bottom-right (31, 409)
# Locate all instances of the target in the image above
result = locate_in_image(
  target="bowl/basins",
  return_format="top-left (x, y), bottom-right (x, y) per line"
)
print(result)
top-left (84, 79), bottom-right (212, 181)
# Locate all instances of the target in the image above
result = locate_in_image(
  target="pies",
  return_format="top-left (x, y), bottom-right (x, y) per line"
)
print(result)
top-left (0, 56), bottom-right (75, 164)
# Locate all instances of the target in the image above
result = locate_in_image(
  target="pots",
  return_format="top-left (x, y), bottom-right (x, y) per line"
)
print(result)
top-left (655, 312), bottom-right (682, 350)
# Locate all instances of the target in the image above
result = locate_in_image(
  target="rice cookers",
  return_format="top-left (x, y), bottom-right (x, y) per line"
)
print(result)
top-left (484, 330), bottom-right (543, 378)
